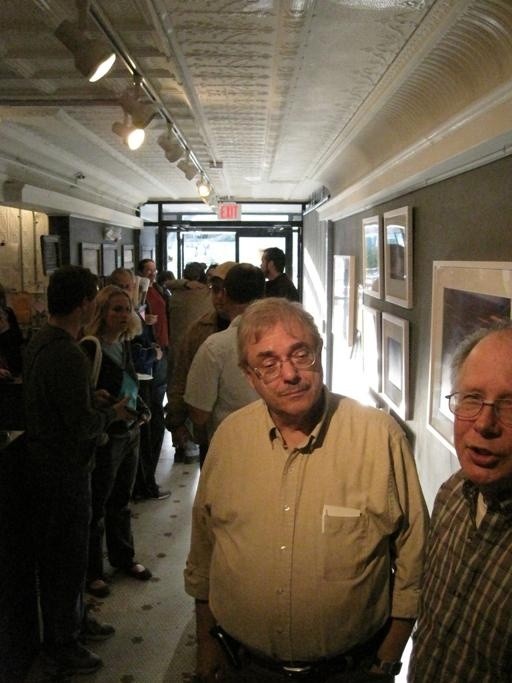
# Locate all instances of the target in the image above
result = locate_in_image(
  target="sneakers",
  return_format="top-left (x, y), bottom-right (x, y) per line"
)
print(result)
top-left (81, 621), bottom-right (115, 641)
top-left (174, 445), bottom-right (200, 464)
top-left (125, 561), bottom-right (151, 581)
top-left (40, 643), bottom-right (102, 673)
top-left (89, 578), bottom-right (109, 597)
top-left (148, 490), bottom-right (171, 500)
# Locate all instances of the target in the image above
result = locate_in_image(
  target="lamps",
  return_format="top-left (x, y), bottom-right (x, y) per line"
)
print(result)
top-left (52, 1), bottom-right (213, 202)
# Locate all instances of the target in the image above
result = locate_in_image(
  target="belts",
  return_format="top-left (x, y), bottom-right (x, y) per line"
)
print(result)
top-left (236, 621), bottom-right (389, 680)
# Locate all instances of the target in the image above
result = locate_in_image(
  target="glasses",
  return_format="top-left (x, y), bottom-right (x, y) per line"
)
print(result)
top-left (208, 285), bottom-right (225, 294)
top-left (445, 391), bottom-right (511, 423)
top-left (245, 342), bottom-right (321, 384)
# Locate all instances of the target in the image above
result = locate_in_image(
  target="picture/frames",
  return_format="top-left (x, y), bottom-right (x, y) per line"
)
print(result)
top-left (41, 234), bottom-right (63, 276)
top-left (328, 201), bottom-right (417, 421)
top-left (121, 244), bottom-right (134, 268)
top-left (426, 260), bottom-right (511, 463)
top-left (101, 244), bottom-right (118, 276)
top-left (81, 241), bottom-right (101, 277)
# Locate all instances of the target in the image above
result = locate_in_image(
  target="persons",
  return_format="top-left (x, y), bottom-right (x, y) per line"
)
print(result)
top-left (136, 258), bottom-right (173, 353)
top-left (77, 284), bottom-right (151, 596)
top-left (262, 248), bottom-right (298, 300)
top-left (0, 286), bottom-right (25, 431)
top-left (109, 268), bottom-right (172, 500)
top-left (181, 296), bottom-right (431, 683)
top-left (23, 264), bottom-right (134, 674)
top-left (157, 271), bottom-right (174, 285)
top-left (166, 263), bottom-right (265, 471)
top-left (405, 316), bottom-right (511, 683)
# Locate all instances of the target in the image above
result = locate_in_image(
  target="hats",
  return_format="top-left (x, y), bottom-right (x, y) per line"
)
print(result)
top-left (207, 262), bottom-right (238, 283)
